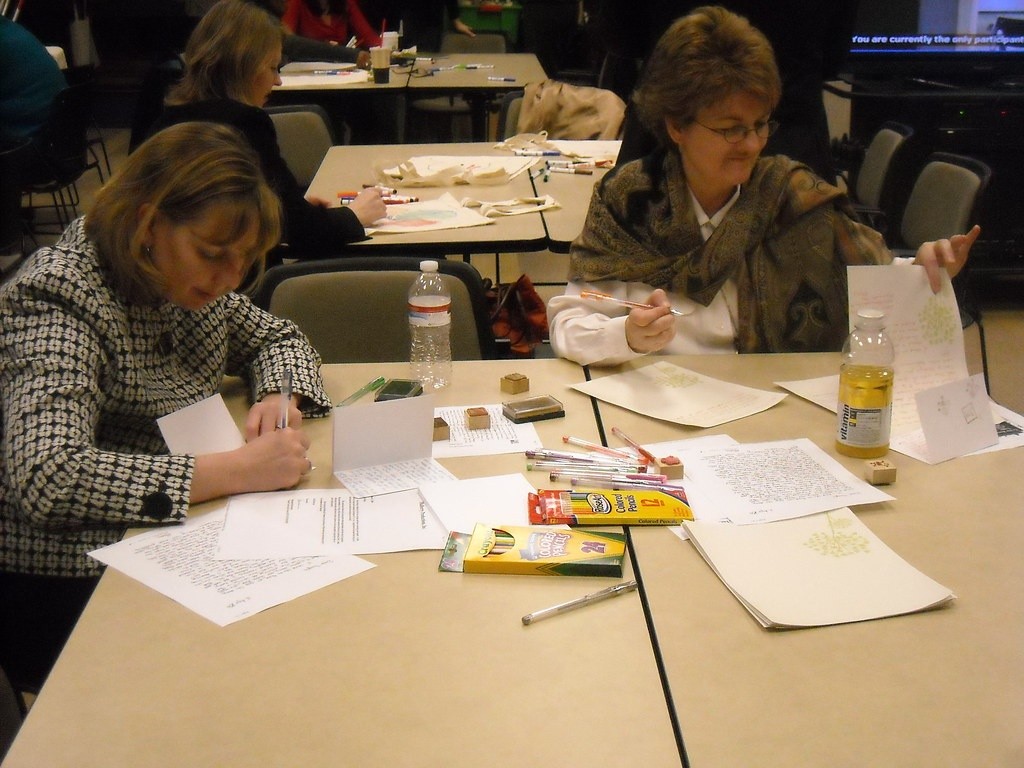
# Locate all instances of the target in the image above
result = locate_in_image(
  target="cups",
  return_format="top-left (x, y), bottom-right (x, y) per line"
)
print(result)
top-left (380, 31), bottom-right (399, 51)
top-left (370, 46), bottom-right (391, 84)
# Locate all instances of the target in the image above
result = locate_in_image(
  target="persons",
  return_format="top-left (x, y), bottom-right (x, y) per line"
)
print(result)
top-left (546, 6), bottom-right (979, 369)
top-left (282, 0), bottom-right (476, 52)
top-left (127, 0), bottom-right (387, 290)
top-left (0, 14), bottom-right (87, 273)
top-left (0, 121), bottom-right (334, 641)
top-left (513, 0), bottom-right (847, 184)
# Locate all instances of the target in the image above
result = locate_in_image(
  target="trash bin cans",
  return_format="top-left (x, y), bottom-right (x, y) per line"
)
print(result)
top-left (452, 4), bottom-right (522, 49)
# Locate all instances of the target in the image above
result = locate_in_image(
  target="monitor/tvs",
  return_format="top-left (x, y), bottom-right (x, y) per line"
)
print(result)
top-left (847, 0), bottom-right (1024, 88)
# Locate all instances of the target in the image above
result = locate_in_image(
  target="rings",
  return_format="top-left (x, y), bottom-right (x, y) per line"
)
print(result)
top-left (276, 425), bottom-right (280, 428)
top-left (304, 457), bottom-right (312, 475)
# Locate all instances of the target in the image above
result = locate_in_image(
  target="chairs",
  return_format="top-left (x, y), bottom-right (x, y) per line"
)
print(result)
top-left (833, 119), bottom-right (998, 397)
top-left (268, 272), bottom-right (481, 365)
top-left (0, 64), bottom-right (114, 279)
top-left (438, 28), bottom-right (507, 118)
top-left (262, 103), bottom-right (340, 190)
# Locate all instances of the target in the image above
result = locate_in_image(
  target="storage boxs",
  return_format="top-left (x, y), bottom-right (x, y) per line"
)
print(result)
top-left (529, 490), bottom-right (694, 525)
top-left (438, 521), bottom-right (627, 577)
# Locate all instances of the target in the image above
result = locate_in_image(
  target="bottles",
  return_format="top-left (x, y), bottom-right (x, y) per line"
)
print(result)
top-left (835, 310), bottom-right (893, 459)
top-left (408, 260), bottom-right (452, 390)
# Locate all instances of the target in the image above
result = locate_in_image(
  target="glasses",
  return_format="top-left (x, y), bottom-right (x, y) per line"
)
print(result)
top-left (694, 120), bottom-right (778, 144)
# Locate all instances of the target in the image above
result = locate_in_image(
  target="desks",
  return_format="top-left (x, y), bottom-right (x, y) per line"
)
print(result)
top-left (408, 50), bottom-right (547, 140)
top-left (271, 56), bottom-right (414, 141)
top-left (531, 141), bottom-right (623, 254)
top-left (303, 143), bottom-right (546, 254)
top-left (4, 359), bottom-right (684, 768)
top-left (587, 353), bottom-right (1023, 768)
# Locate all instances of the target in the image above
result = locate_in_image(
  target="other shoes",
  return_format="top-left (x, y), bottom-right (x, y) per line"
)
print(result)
top-left (0, 232), bottom-right (25, 257)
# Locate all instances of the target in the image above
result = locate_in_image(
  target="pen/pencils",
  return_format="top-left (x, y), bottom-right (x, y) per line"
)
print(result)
top-left (529, 168), bottom-right (545, 180)
top-left (522, 581), bottom-right (638, 626)
top-left (337, 191), bottom-right (419, 205)
top-left (542, 170), bottom-right (550, 182)
top-left (528, 459), bottom-right (686, 498)
top-left (416, 57), bottom-right (433, 61)
top-left (312, 70), bottom-right (339, 74)
top-left (546, 159), bottom-right (613, 175)
top-left (362, 184), bottom-right (397, 194)
top-left (525, 450), bottom-right (610, 464)
top-left (278, 368), bottom-right (293, 430)
top-left (334, 376), bottom-right (384, 407)
top-left (515, 150), bottom-right (562, 156)
top-left (488, 76), bottom-right (516, 83)
top-left (454, 64), bottom-right (496, 70)
top-left (580, 290), bottom-right (685, 317)
top-left (561, 436), bottom-right (638, 460)
top-left (326, 71), bottom-right (350, 74)
top-left (613, 426), bottom-right (656, 463)
